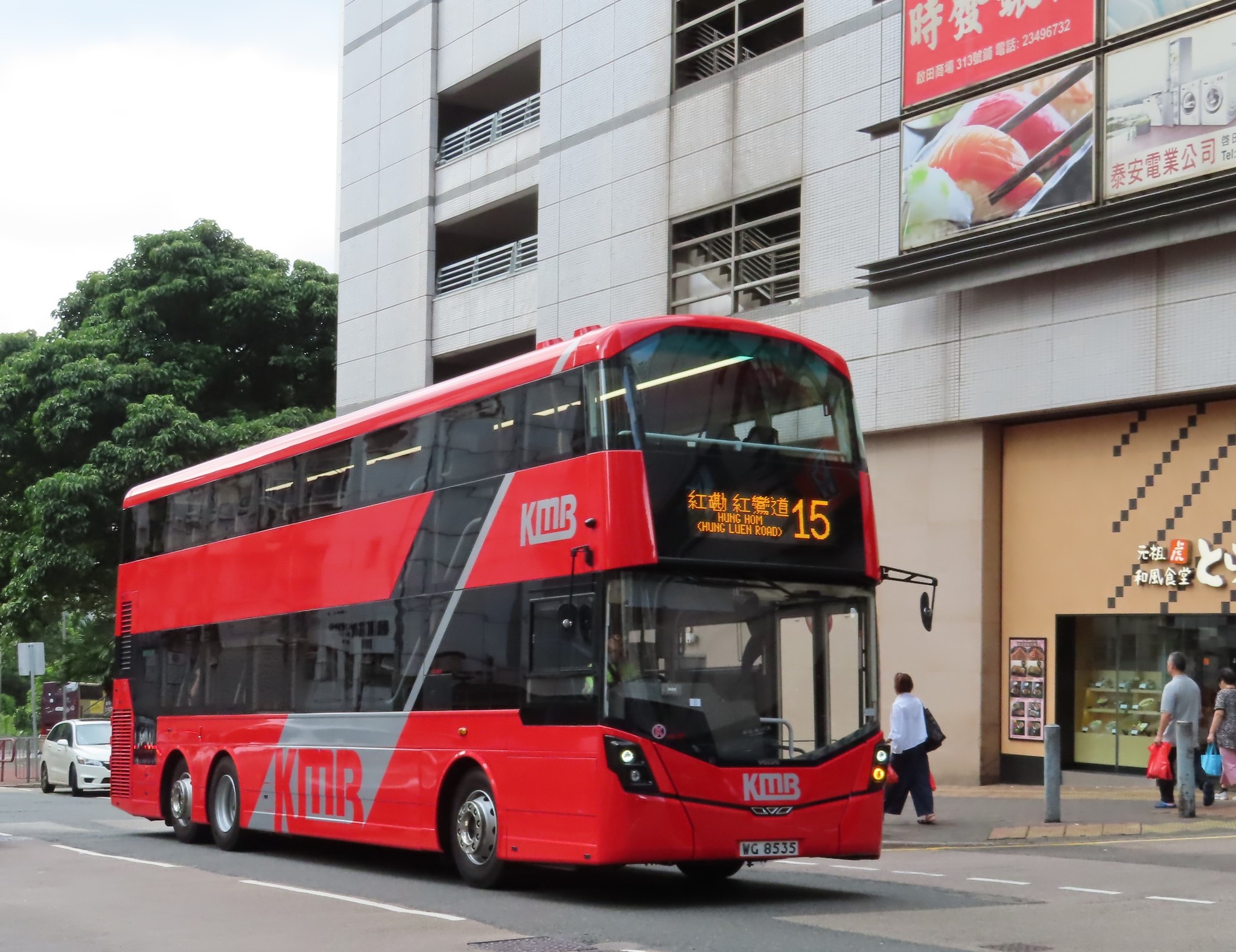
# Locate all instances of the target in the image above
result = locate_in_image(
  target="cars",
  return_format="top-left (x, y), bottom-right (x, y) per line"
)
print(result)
top-left (40, 718), bottom-right (112, 796)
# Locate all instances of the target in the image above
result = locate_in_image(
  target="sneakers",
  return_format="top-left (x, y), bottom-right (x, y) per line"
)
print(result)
top-left (1214, 791), bottom-right (1229, 800)
top-left (1232, 796), bottom-right (1236, 800)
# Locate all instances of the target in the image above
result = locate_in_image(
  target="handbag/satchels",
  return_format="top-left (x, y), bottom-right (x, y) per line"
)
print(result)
top-left (921, 703), bottom-right (946, 753)
top-left (1201, 742), bottom-right (1224, 777)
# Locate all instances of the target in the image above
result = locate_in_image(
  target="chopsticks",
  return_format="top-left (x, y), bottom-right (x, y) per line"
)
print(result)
top-left (987, 60), bottom-right (1093, 205)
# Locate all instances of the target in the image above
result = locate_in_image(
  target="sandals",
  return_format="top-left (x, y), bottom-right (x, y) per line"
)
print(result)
top-left (917, 814), bottom-right (937, 824)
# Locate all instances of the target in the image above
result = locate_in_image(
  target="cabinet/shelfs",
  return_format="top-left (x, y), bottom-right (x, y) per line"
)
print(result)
top-left (1073, 615), bottom-right (1170, 768)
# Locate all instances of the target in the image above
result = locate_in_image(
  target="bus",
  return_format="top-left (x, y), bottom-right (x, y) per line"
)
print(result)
top-left (109, 310), bottom-right (940, 891)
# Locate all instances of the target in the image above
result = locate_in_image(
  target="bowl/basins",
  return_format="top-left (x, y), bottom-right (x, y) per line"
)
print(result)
top-left (902, 114), bottom-right (951, 140)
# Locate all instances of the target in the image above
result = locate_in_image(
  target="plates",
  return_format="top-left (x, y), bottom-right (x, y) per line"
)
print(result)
top-left (910, 101), bottom-right (1093, 221)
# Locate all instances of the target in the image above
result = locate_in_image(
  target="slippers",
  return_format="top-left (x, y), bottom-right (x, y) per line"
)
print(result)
top-left (1203, 780), bottom-right (1214, 807)
top-left (1155, 802), bottom-right (1177, 808)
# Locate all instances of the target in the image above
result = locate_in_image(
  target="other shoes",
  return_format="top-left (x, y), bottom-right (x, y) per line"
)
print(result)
top-left (1146, 741), bottom-right (1173, 781)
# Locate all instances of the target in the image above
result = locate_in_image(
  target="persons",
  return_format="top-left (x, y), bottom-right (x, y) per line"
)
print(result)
top-left (706, 410), bottom-right (777, 444)
top-left (886, 672), bottom-right (947, 824)
top-left (1147, 652), bottom-right (1215, 807)
top-left (582, 627), bottom-right (647, 695)
top-left (1207, 671), bottom-right (1236, 801)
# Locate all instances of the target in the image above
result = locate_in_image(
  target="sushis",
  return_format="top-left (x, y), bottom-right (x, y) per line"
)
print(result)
top-left (899, 65), bottom-right (1097, 252)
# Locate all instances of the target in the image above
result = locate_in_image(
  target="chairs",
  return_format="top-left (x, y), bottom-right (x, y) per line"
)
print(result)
top-left (168, 419), bottom-right (777, 549)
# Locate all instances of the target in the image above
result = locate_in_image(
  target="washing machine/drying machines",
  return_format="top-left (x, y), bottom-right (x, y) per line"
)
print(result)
top-left (1201, 71), bottom-right (1236, 125)
top-left (1179, 79), bottom-right (1201, 125)
